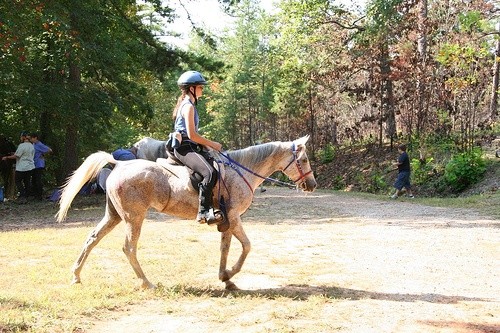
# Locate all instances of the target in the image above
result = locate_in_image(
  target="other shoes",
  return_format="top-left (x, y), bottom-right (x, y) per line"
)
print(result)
top-left (35, 199), bottom-right (44, 206)
top-left (405, 195), bottom-right (414, 199)
top-left (390, 194), bottom-right (398, 199)
top-left (80, 186), bottom-right (88, 195)
top-left (26, 196), bottom-right (35, 201)
top-left (13, 197), bottom-right (27, 205)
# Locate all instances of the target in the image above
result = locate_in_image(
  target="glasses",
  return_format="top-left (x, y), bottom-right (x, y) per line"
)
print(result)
top-left (194, 85), bottom-right (203, 89)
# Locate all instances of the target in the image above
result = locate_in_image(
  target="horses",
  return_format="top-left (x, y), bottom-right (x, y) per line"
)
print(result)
top-left (54, 133), bottom-right (317, 290)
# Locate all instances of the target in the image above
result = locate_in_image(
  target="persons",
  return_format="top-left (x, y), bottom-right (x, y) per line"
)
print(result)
top-left (390, 144), bottom-right (414, 198)
top-left (0, 129), bottom-right (35, 204)
top-left (172, 70), bottom-right (226, 224)
top-left (30, 133), bottom-right (52, 200)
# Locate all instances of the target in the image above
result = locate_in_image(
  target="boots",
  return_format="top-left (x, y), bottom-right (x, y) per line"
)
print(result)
top-left (196, 183), bottom-right (222, 222)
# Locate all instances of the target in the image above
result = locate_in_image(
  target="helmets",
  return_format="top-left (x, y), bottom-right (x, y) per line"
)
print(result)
top-left (177, 70), bottom-right (208, 86)
